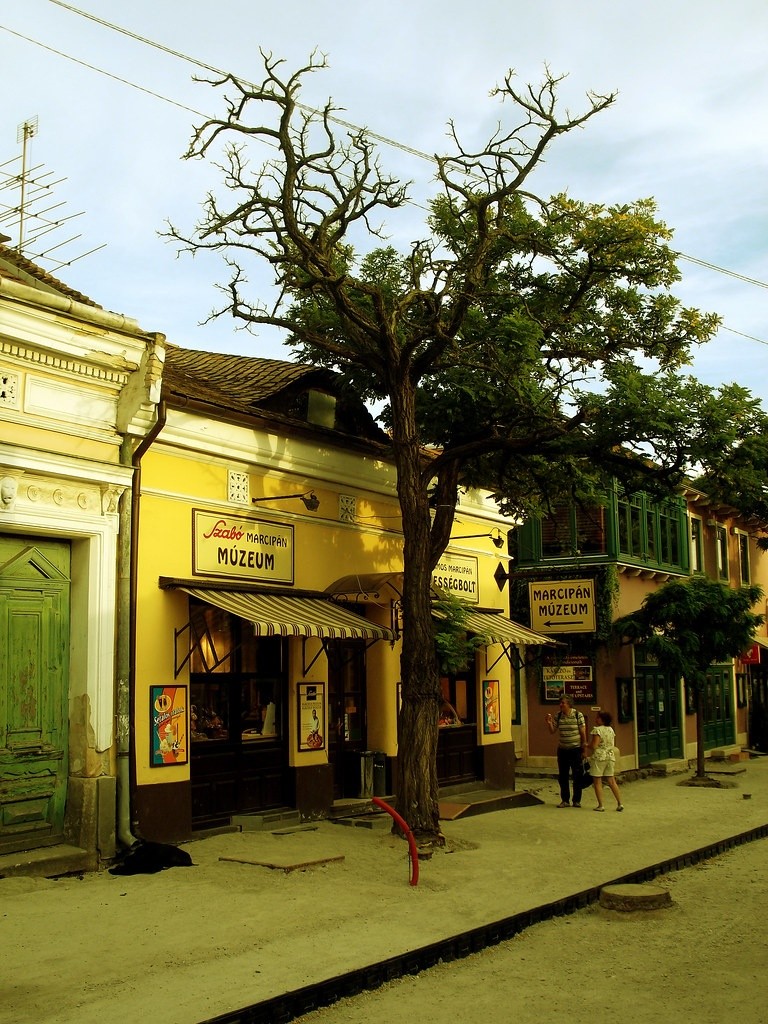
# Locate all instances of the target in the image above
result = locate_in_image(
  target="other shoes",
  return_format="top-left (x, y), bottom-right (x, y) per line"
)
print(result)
top-left (557, 801), bottom-right (570, 808)
top-left (616, 805), bottom-right (623, 811)
top-left (573, 802), bottom-right (581, 806)
top-left (593, 806), bottom-right (604, 811)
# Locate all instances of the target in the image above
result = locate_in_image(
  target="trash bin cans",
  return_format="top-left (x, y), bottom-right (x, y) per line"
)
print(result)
top-left (358, 750), bottom-right (387, 799)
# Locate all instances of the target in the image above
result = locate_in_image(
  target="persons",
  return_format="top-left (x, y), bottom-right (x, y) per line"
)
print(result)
top-left (310, 710), bottom-right (319, 738)
top-left (546, 695), bottom-right (588, 808)
top-left (588, 711), bottom-right (624, 812)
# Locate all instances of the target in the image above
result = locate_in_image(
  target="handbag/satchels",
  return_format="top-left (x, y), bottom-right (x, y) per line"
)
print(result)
top-left (584, 758), bottom-right (590, 771)
top-left (580, 757), bottom-right (593, 790)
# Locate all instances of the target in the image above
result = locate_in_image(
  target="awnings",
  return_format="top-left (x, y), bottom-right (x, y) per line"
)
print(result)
top-left (173, 588), bottom-right (397, 679)
top-left (432, 610), bottom-right (561, 674)
